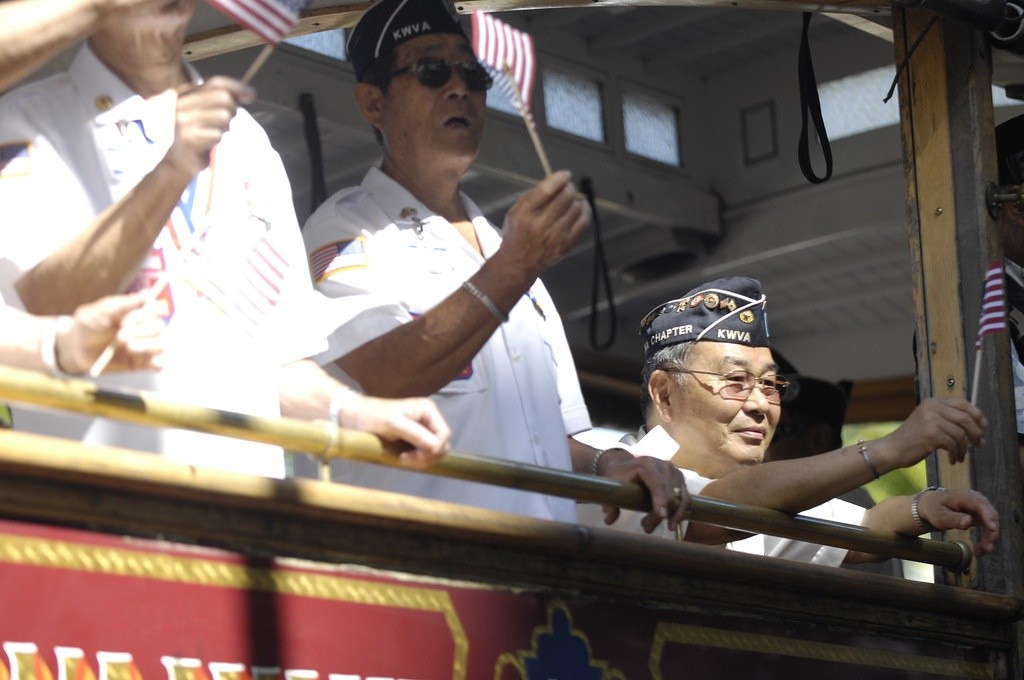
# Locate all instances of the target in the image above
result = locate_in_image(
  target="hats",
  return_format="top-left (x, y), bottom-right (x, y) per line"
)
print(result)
top-left (344, 0), bottom-right (472, 82)
top-left (639, 277), bottom-right (770, 359)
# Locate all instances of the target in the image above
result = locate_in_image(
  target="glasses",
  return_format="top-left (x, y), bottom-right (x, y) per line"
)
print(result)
top-left (664, 368), bottom-right (793, 403)
top-left (375, 56), bottom-right (494, 91)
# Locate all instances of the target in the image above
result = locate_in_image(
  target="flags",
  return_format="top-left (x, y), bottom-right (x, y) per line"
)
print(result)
top-left (473, 13), bottom-right (533, 115)
top-left (215, 2), bottom-right (304, 47)
top-left (977, 260), bottom-right (1023, 367)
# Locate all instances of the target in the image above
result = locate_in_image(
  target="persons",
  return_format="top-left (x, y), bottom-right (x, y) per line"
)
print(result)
top-left (580, 278), bottom-right (999, 563)
top-left (0, 0), bottom-right (692, 536)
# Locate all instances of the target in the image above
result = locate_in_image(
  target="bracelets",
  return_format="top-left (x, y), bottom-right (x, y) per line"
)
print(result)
top-left (462, 280), bottom-right (510, 325)
top-left (329, 383), bottom-right (356, 429)
top-left (42, 313), bottom-right (86, 382)
top-left (858, 440), bottom-right (881, 481)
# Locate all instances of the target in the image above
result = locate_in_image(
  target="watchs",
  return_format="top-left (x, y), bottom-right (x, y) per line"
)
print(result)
top-left (592, 446), bottom-right (633, 476)
top-left (912, 486), bottom-right (951, 533)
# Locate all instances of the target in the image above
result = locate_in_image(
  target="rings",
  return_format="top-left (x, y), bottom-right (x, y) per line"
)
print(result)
top-left (673, 486), bottom-right (683, 498)
